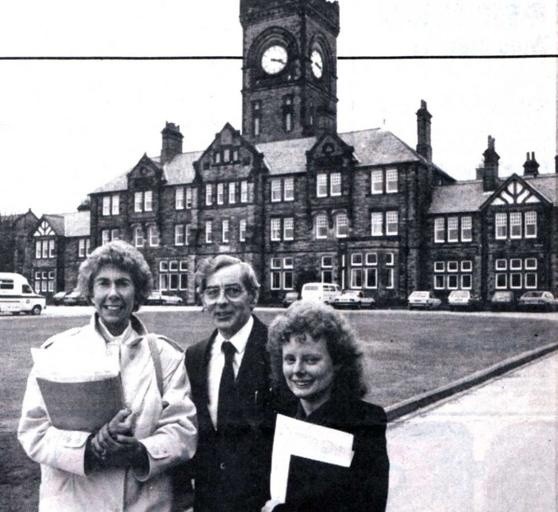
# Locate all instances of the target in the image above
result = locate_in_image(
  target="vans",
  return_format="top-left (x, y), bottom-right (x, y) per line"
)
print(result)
top-left (0, 271), bottom-right (46, 315)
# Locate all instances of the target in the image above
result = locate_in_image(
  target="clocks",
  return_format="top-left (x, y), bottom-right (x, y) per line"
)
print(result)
top-left (259, 43), bottom-right (289, 77)
top-left (309, 48), bottom-right (325, 79)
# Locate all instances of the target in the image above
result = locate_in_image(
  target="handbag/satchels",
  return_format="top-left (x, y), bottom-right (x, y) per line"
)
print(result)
top-left (169, 461), bottom-right (194, 512)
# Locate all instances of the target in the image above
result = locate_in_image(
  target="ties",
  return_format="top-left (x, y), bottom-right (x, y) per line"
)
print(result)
top-left (217, 341), bottom-right (237, 431)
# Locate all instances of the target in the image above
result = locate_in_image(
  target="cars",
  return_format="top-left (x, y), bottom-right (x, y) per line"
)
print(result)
top-left (145, 290), bottom-right (183, 306)
top-left (53, 291), bottom-right (89, 306)
top-left (281, 282), bottom-right (376, 309)
top-left (407, 290), bottom-right (558, 310)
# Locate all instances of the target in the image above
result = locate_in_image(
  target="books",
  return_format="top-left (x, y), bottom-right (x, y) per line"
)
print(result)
top-left (35, 370), bottom-right (126, 434)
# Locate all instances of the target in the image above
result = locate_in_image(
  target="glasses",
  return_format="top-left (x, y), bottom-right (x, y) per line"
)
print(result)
top-left (203, 288), bottom-right (245, 298)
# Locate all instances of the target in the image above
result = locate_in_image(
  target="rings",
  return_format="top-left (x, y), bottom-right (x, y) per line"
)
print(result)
top-left (98, 449), bottom-right (107, 457)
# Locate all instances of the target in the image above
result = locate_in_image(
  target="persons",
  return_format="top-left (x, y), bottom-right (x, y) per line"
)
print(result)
top-left (15, 236), bottom-right (200, 511)
top-left (172, 253), bottom-right (297, 511)
top-left (230, 296), bottom-right (391, 511)
